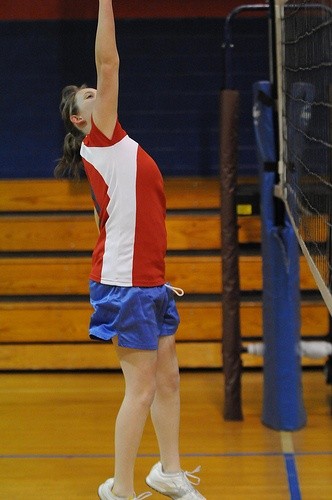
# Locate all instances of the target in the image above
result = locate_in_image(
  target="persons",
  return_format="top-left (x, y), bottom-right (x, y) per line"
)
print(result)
top-left (53, 0), bottom-right (209, 500)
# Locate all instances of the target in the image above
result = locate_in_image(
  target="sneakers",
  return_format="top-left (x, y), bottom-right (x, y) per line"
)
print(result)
top-left (98, 477), bottom-right (152, 500)
top-left (145, 461), bottom-right (207, 500)
